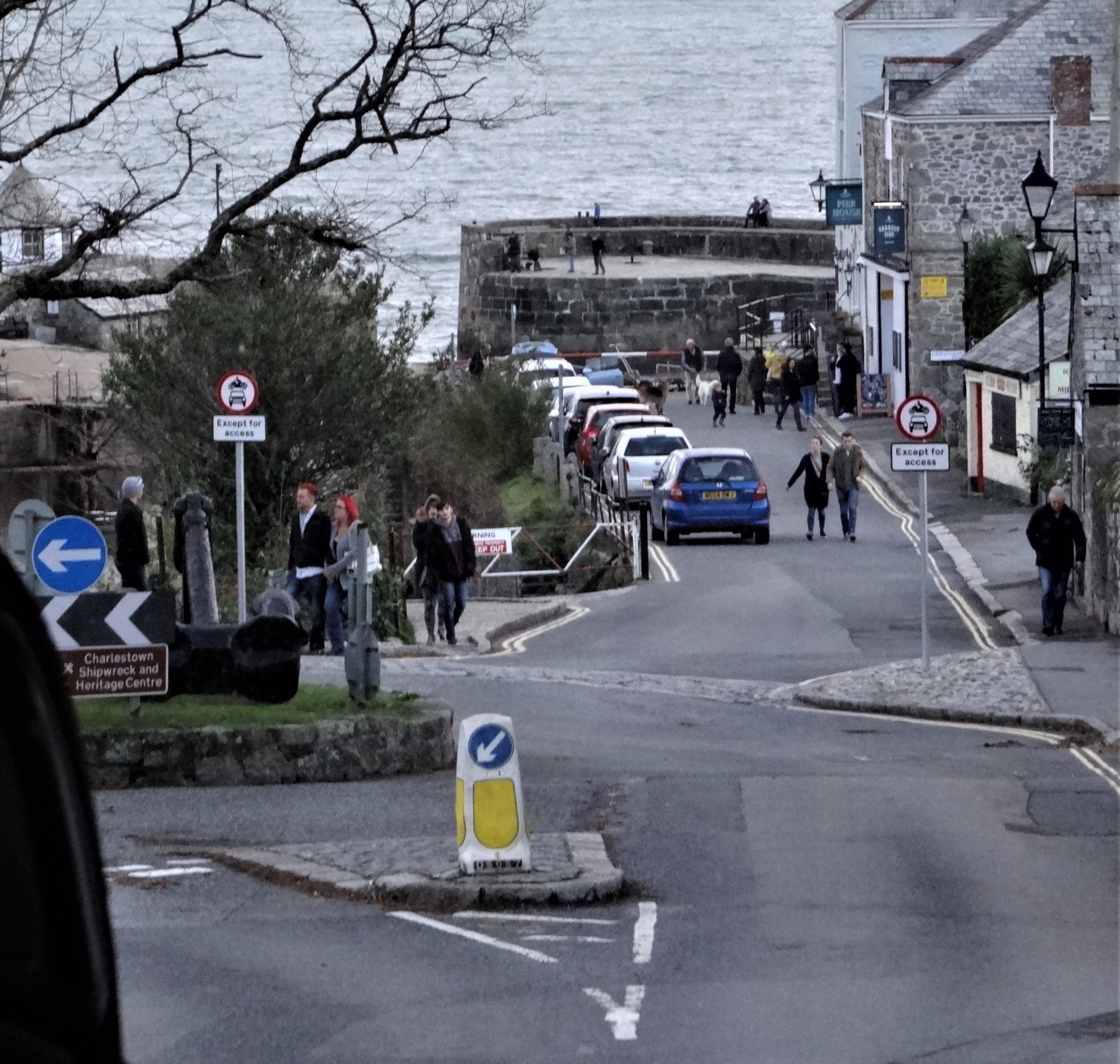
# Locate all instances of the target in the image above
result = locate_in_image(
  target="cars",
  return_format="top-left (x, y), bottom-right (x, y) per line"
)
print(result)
top-left (647, 449), bottom-right (772, 546)
top-left (502, 342), bottom-right (691, 509)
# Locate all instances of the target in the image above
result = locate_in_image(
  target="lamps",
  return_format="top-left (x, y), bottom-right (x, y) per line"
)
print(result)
top-left (810, 168), bottom-right (830, 212)
top-left (1022, 148), bottom-right (1076, 237)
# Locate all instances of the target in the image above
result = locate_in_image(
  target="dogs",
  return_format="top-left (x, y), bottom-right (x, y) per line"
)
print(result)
top-left (637, 376), bottom-right (670, 414)
top-left (696, 373), bottom-right (721, 405)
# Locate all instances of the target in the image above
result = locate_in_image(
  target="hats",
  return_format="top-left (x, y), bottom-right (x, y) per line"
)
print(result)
top-left (777, 346), bottom-right (788, 352)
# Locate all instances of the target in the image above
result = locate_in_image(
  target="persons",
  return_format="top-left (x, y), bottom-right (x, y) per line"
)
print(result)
top-left (776, 358), bottom-right (807, 431)
top-left (785, 436), bottom-right (831, 539)
top-left (591, 236), bottom-right (605, 276)
top-left (284, 485), bottom-right (331, 658)
top-left (801, 345), bottom-right (820, 420)
top-left (829, 342), bottom-right (857, 420)
top-left (116, 475), bottom-right (152, 591)
top-left (469, 343), bottom-right (492, 375)
top-left (716, 337), bottom-right (743, 413)
top-left (831, 430), bottom-right (867, 542)
top-left (747, 348), bottom-right (767, 415)
top-left (744, 196), bottom-right (771, 229)
top-left (504, 232), bottom-right (540, 273)
top-left (413, 494), bottom-right (476, 646)
top-left (711, 380), bottom-right (725, 428)
top-left (593, 200), bottom-right (600, 229)
top-left (565, 230), bottom-right (577, 276)
top-left (1027, 486), bottom-right (1087, 639)
top-left (325, 495), bottom-right (370, 657)
top-left (681, 338), bottom-right (706, 406)
top-left (174, 492), bottom-right (213, 625)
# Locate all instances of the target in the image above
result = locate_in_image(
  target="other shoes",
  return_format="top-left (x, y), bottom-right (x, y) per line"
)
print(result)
top-left (761, 409), bottom-right (766, 413)
top-left (696, 400), bottom-right (700, 404)
top-left (753, 412), bottom-right (760, 416)
top-left (844, 532), bottom-right (850, 539)
top-left (688, 400), bottom-right (692, 405)
top-left (568, 269), bottom-right (575, 273)
top-left (806, 532), bottom-right (812, 541)
top-left (592, 271), bottom-right (606, 276)
top-left (820, 533), bottom-right (826, 539)
top-left (729, 411), bottom-right (737, 414)
top-left (849, 532), bottom-right (857, 542)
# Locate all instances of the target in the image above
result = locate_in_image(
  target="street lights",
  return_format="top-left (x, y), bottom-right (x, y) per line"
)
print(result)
top-left (953, 200), bottom-right (977, 398)
top-left (1020, 151), bottom-right (1058, 417)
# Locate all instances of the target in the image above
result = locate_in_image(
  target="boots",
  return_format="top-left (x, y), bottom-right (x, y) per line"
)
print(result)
top-left (713, 420), bottom-right (718, 427)
top-left (796, 423), bottom-right (807, 432)
top-left (775, 421), bottom-right (783, 430)
top-left (719, 419), bottom-right (725, 427)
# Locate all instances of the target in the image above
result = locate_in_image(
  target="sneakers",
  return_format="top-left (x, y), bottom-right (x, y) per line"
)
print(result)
top-left (850, 413), bottom-right (853, 418)
top-left (838, 412), bottom-right (851, 420)
